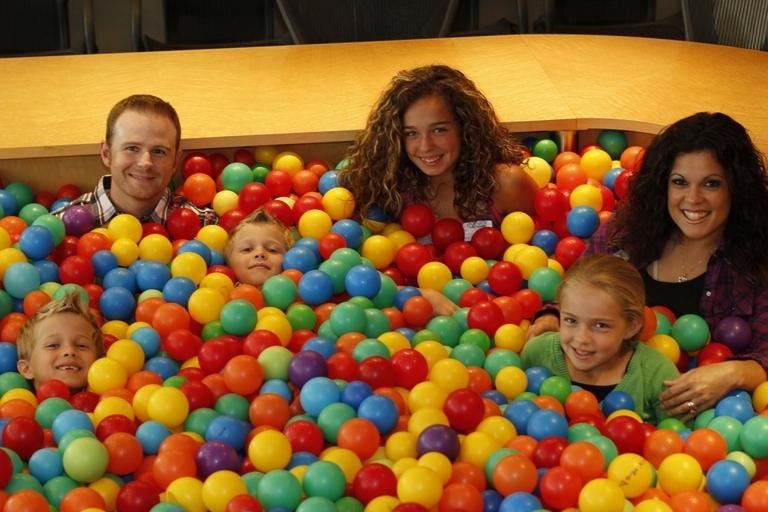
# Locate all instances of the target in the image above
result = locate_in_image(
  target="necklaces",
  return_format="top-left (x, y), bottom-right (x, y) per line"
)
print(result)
top-left (677, 235), bottom-right (717, 283)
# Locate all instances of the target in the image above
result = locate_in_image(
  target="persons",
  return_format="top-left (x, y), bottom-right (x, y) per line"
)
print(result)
top-left (48, 95), bottom-right (221, 230)
top-left (222, 207), bottom-right (295, 292)
top-left (336, 63), bottom-right (541, 244)
top-left (573, 112), bottom-right (767, 426)
top-left (521, 253), bottom-right (694, 432)
top-left (17, 288), bottom-right (107, 397)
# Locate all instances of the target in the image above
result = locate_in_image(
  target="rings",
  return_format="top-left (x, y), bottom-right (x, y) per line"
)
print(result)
top-left (687, 401), bottom-right (695, 409)
